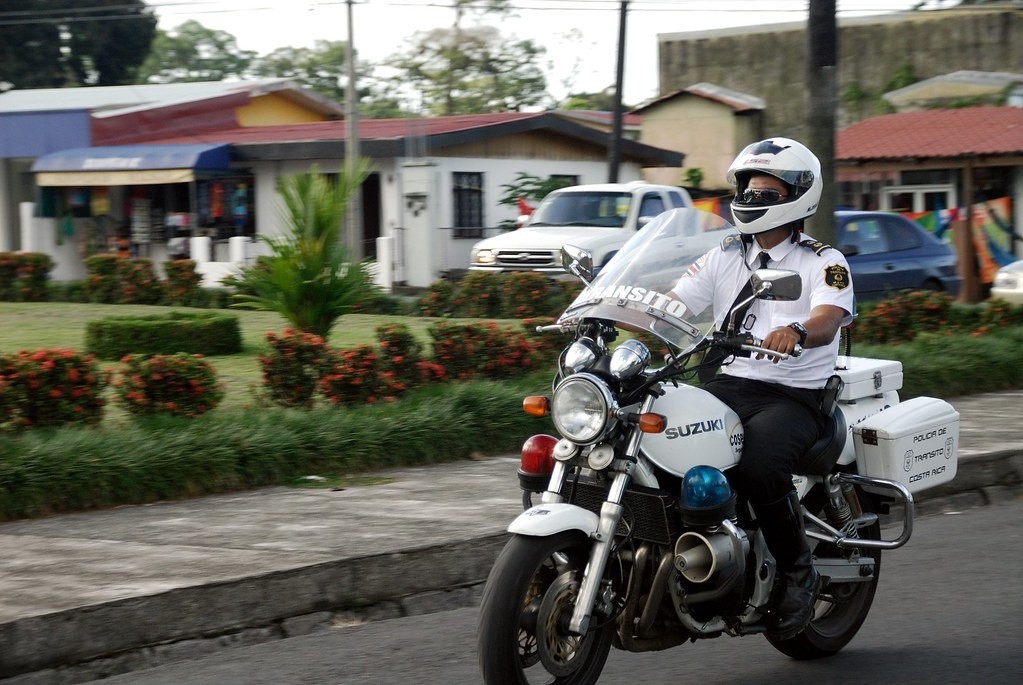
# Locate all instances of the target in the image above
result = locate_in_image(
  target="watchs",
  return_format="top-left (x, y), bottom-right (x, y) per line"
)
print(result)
top-left (785, 322), bottom-right (808, 349)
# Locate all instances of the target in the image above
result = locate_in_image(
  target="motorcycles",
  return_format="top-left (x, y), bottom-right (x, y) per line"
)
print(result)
top-left (475, 249), bottom-right (963, 685)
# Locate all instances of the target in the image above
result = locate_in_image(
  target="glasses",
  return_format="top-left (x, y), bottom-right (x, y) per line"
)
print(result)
top-left (743, 188), bottom-right (791, 202)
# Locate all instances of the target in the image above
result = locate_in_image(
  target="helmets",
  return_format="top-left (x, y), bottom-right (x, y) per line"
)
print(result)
top-left (726, 137), bottom-right (824, 235)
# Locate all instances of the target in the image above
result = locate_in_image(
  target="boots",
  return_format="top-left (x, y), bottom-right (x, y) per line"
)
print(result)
top-left (756, 489), bottom-right (820, 641)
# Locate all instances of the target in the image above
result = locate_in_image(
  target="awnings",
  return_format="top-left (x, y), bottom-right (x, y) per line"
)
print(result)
top-left (28, 143), bottom-right (230, 187)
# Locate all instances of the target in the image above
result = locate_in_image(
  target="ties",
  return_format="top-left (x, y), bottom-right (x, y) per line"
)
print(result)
top-left (698, 244), bottom-right (771, 383)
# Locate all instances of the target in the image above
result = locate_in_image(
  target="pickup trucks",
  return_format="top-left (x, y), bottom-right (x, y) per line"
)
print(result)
top-left (469, 183), bottom-right (696, 280)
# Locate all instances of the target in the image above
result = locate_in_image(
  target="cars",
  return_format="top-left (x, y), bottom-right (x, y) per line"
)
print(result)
top-left (832, 209), bottom-right (966, 305)
top-left (990, 259), bottom-right (1023, 318)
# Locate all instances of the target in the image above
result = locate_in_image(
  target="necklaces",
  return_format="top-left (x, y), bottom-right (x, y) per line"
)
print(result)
top-left (743, 253), bottom-right (789, 330)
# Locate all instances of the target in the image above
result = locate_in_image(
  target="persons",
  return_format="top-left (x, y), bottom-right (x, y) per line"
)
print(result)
top-left (559, 136), bottom-right (854, 642)
